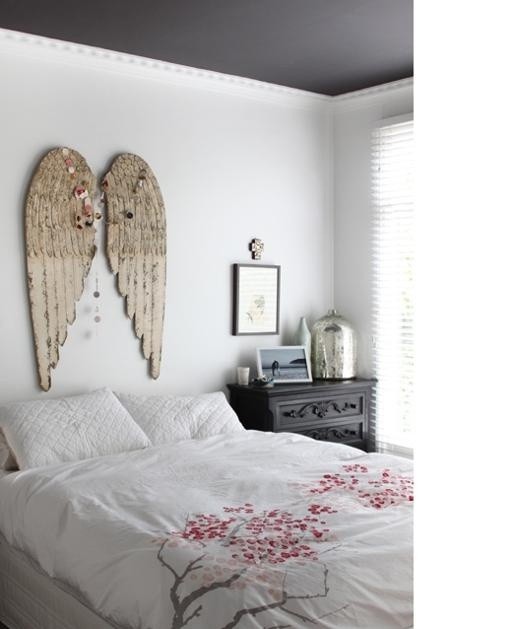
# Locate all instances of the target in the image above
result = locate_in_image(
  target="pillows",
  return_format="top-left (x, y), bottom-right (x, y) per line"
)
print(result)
top-left (0, 389), bottom-right (245, 473)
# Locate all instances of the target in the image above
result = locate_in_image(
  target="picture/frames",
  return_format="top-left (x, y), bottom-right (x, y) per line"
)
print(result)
top-left (233, 263), bottom-right (314, 386)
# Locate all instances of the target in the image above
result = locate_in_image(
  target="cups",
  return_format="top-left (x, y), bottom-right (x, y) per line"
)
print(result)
top-left (237, 367), bottom-right (249, 386)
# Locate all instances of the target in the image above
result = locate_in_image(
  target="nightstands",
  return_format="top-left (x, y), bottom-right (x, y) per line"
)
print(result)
top-left (227, 379), bottom-right (378, 451)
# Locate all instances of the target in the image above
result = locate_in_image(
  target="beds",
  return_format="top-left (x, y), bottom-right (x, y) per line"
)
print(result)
top-left (0, 428), bottom-right (414, 629)
